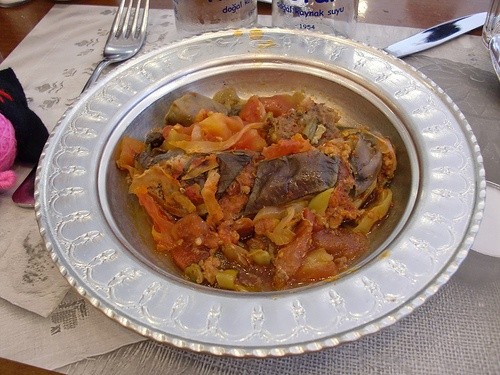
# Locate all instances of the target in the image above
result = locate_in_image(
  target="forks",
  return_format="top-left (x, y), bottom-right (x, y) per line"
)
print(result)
top-left (14, 0), bottom-right (150, 208)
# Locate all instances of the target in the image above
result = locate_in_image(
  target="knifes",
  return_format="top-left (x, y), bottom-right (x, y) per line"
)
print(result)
top-left (380, 8), bottom-right (489, 57)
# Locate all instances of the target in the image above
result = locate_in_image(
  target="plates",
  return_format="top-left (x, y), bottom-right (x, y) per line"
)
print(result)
top-left (31, 24), bottom-right (488, 360)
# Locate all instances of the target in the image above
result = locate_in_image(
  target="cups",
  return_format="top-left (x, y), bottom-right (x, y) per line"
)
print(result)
top-left (482, 0), bottom-right (500, 48)
top-left (270, 0), bottom-right (356, 40)
top-left (171, 0), bottom-right (259, 37)
